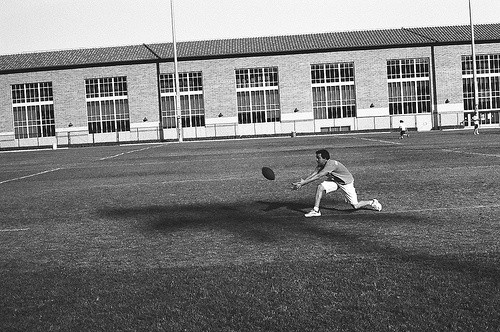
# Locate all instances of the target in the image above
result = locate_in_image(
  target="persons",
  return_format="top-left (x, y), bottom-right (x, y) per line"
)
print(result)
top-left (399, 120), bottom-right (410, 139)
top-left (472, 112), bottom-right (481, 135)
top-left (290, 149), bottom-right (382, 217)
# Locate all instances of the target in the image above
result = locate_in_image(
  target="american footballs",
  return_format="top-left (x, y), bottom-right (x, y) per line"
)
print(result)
top-left (262, 167), bottom-right (275, 181)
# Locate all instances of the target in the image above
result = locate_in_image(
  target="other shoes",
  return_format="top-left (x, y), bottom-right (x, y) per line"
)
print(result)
top-left (477, 133), bottom-right (479, 134)
top-left (398, 136), bottom-right (403, 139)
top-left (473, 133), bottom-right (476, 135)
top-left (407, 134), bottom-right (409, 138)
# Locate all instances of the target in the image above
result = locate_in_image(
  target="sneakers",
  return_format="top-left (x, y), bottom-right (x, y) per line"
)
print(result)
top-left (371, 198), bottom-right (382, 212)
top-left (304, 209), bottom-right (322, 217)
